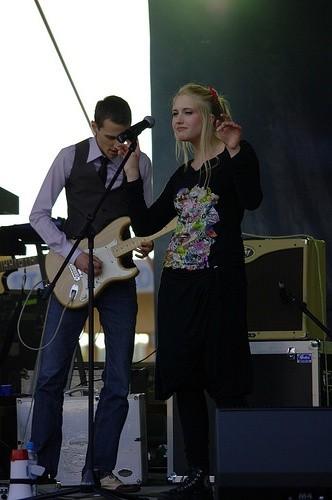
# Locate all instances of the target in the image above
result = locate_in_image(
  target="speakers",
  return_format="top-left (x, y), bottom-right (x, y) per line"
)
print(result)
top-left (243, 239), bottom-right (328, 341)
top-left (212, 407), bottom-right (332, 500)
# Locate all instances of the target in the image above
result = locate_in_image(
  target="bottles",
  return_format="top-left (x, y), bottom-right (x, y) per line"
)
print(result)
top-left (22, 441), bottom-right (37, 496)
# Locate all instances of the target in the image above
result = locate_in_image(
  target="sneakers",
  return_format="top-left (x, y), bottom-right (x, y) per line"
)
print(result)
top-left (158, 469), bottom-right (214, 500)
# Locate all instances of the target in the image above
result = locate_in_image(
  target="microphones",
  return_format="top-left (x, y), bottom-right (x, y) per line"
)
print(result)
top-left (116, 116), bottom-right (155, 143)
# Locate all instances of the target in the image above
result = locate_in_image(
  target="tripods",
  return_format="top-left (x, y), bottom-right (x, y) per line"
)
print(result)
top-left (30, 137), bottom-right (146, 500)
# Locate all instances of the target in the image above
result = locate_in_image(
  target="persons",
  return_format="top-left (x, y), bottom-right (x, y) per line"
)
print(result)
top-left (114, 83), bottom-right (263, 500)
top-left (26, 96), bottom-right (153, 490)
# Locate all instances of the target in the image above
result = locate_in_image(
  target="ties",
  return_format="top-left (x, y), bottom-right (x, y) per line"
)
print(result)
top-left (97, 155), bottom-right (113, 185)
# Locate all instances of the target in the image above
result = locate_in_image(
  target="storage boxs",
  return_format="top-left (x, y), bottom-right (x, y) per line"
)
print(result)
top-left (16, 392), bottom-right (149, 485)
top-left (166, 340), bottom-right (332, 483)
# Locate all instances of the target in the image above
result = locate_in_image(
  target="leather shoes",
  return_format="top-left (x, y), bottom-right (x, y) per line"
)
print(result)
top-left (93, 469), bottom-right (141, 493)
top-left (31, 475), bottom-right (72, 495)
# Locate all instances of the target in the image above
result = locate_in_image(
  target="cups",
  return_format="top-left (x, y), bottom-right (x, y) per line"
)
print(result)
top-left (1, 384), bottom-right (11, 396)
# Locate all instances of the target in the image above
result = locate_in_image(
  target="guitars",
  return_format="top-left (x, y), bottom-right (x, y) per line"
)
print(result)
top-left (46, 218), bottom-right (181, 309)
